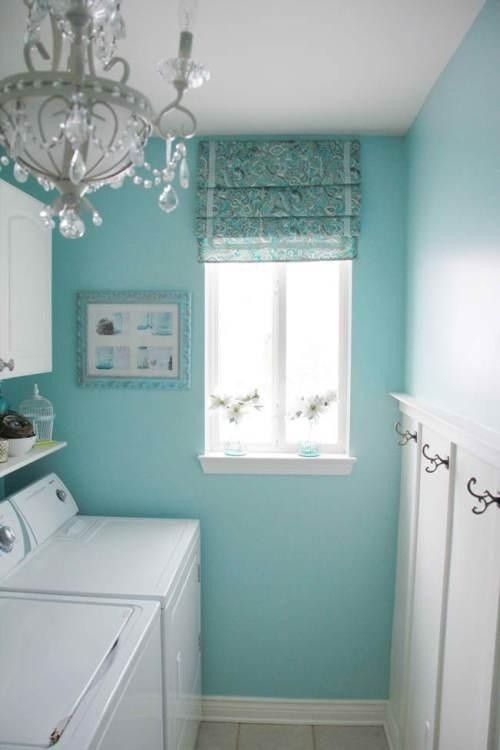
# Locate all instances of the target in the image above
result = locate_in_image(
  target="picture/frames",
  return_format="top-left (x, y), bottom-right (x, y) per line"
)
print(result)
top-left (74, 287), bottom-right (192, 393)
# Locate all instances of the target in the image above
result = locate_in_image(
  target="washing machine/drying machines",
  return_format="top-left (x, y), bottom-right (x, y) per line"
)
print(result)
top-left (0, 592), bottom-right (166, 750)
top-left (1, 472), bottom-right (202, 749)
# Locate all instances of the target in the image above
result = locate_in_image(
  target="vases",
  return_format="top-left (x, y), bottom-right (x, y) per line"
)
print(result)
top-left (225, 424), bottom-right (247, 456)
top-left (299, 421), bottom-right (322, 458)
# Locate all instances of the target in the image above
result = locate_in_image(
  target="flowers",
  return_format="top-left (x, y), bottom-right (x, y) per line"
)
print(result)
top-left (206, 388), bottom-right (264, 424)
top-left (291, 388), bottom-right (337, 422)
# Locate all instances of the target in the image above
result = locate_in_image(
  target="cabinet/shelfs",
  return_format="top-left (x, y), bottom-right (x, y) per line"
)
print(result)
top-left (0, 179), bottom-right (54, 384)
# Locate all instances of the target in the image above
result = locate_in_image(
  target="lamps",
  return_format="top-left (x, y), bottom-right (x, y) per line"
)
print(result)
top-left (0, 0), bottom-right (209, 244)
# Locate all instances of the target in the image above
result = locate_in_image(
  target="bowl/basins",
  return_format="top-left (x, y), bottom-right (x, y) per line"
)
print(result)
top-left (1, 434), bottom-right (38, 458)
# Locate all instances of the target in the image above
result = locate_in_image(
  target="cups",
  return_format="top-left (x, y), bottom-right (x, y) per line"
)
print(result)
top-left (0, 437), bottom-right (9, 464)
